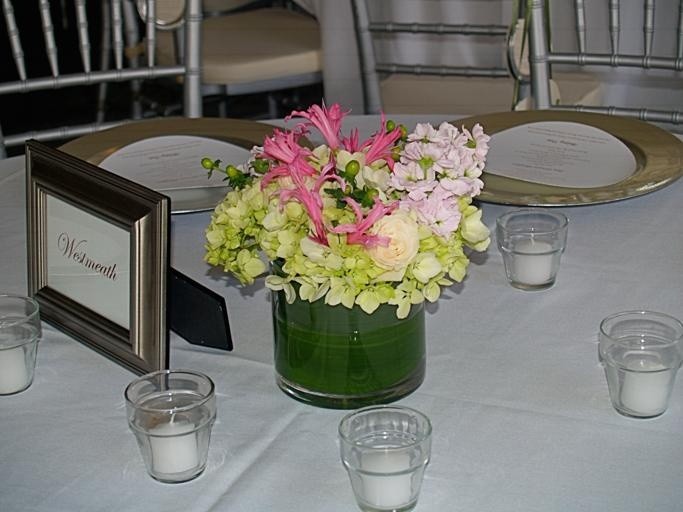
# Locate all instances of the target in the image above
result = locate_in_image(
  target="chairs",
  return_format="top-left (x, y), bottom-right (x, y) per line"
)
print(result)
top-left (0, 0), bottom-right (682, 152)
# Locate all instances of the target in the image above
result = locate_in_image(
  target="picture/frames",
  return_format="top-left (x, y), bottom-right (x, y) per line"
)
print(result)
top-left (20, 135), bottom-right (168, 392)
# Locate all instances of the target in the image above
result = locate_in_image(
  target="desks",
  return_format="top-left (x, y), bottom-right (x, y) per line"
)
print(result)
top-left (1, 108), bottom-right (683, 512)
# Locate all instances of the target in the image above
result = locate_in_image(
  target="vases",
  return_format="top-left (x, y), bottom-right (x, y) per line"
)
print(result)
top-left (266, 259), bottom-right (426, 412)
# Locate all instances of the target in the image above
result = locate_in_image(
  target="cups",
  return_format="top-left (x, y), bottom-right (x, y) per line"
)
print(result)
top-left (0, 292), bottom-right (42, 397)
top-left (495, 208), bottom-right (571, 291)
top-left (598, 309), bottom-right (683, 419)
top-left (337, 404), bottom-right (434, 511)
top-left (123, 370), bottom-right (220, 484)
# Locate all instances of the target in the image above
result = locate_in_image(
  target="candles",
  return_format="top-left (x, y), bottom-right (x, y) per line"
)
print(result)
top-left (1, 341), bottom-right (27, 396)
top-left (619, 359), bottom-right (669, 415)
top-left (514, 240), bottom-right (552, 284)
top-left (150, 422), bottom-right (199, 476)
top-left (359, 443), bottom-right (412, 508)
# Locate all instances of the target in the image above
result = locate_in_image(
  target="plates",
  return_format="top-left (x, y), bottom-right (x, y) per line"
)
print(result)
top-left (55, 117), bottom-right (315, 215)
top-left (431, 110), bottom-right (683, 208)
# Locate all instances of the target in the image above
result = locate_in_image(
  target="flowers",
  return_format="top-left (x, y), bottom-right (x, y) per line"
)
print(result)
top-left (192, 96), bottom-right (492, 319)
